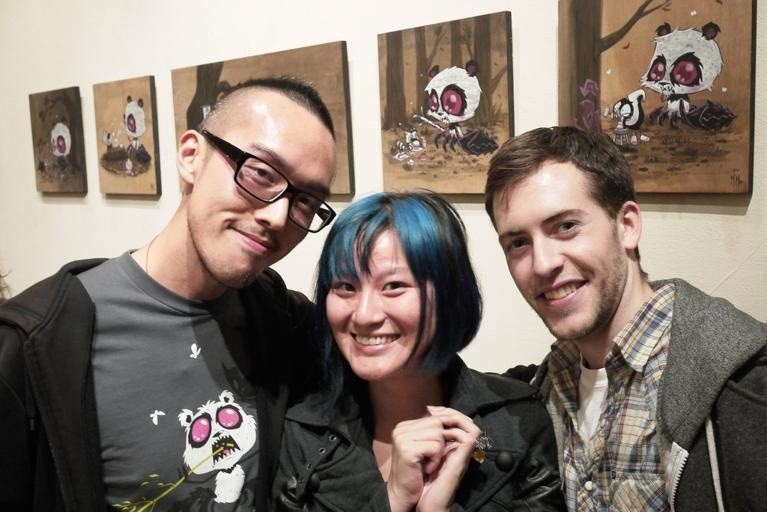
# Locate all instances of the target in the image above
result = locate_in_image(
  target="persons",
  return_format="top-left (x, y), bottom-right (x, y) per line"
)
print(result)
top-left (265, 188), bottom-right (569, 511)
top-left (483, 126), bottom-right (764, 511)
top-left (2, 72), bottom-right (337, 511)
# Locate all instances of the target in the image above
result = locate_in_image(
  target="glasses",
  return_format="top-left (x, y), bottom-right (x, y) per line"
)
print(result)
top-left (200, 129), bottom-right (338, 235)
